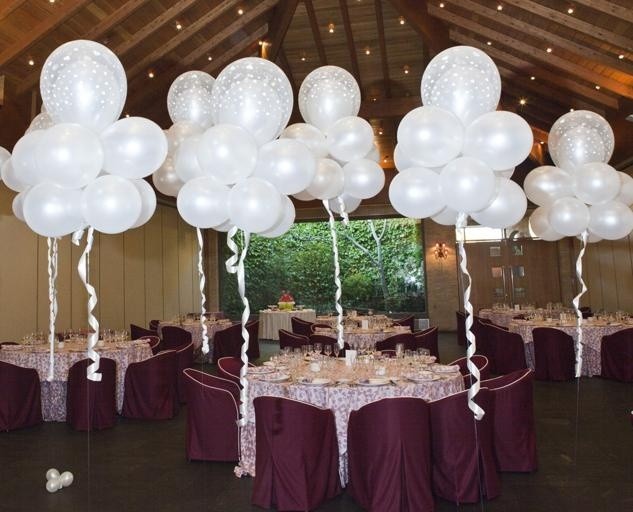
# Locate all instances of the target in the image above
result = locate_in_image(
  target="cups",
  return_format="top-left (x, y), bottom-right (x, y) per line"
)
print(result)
top-left (58, 342), bottom-right (64, 349)
top-left (405, 349), bottom-right (413, 360)
top-left (423, 349), bottom-right (431, 363)
top-left (97, 339), bottom-right (104, 347)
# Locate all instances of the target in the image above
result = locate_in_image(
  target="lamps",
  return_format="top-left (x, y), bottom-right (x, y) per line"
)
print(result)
top-left (434, 239), bottom-right (447, 260)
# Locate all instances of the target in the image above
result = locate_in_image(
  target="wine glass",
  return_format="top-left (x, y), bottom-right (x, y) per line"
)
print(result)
top-left (270, 355), bottom-right (280, 373)
top-left (396, 343), bottom-right (404, 360)
top-left (282, 344), bottom-right (307, 371)
top-left (62, 325), bottom-right (87, 350)
top-left (334, 343), bottom-right (340, 364)
top-left (307, 344), bottom-right (313, 357)
top-left (104, 327), bottom-right (133, 348)
top-left (23, 329), bottom-right (47, 348)
top-left (313, 342), bottom-right (323, 356)
top-left (417, 348), bottom-right (424, 362)
top-left (324, 345), bottom-right (333, 357)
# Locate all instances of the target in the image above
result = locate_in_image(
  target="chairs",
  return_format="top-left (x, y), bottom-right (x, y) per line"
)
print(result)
top-left (447, 354), bottom-right (491, 388)
top-left (466, 313), bottom-right (491, 350)
top-left (160, 325), bottom-right (194, 405)
top-left (150, 319), bottom-right (159, 330)
top-left (66, 357), bottom-right (115, 429)
top-left (290, 316), bottom-right (314, 334)
top-left (121, 349), bottom-right (182, 420)
top-left (278, 327), bottom-right (309, 353)
top-left (0, 360), bottom-right (44, 431)
top-left (245, 319), bottom-right (260, 358)
top-left (473, 367), bottom-right (537, 472)
top-left (427, 388), bottom-right (501, 503)
top-left (397, 313), bottom-right (414, 331)
top-left (347, 398), bottom-right (436, 511)
top-left (415, 325), bottom-right (439, 358)
top-left (182, 367), bottom-right (241, 462)
top-left (578, 306), bottom-right (593, 318)
top-left (137, 335), bottom-right (160, 355)
top-left (217, 356), bottom-right (256, 389)
top-left (213, 324), bottom-right (242, 366)
top-left (601, 328), bottom-right (633, 382)
top-left (456, 310), bottom-right (466, 346)
top-left (478, 319), bottom-right (527, 374)
top-left (252, 397), bottom-right (343, 512)
top-left (311, 323), bottom-right (331, 334)
top-left (532, 326), bottom-right (576, 385)
top-left (130, 323), bottom-right (158, 339)
top-left (310, 334), bottom-right (349, 358)
top-left (377, 333), bottom-right (416, 352)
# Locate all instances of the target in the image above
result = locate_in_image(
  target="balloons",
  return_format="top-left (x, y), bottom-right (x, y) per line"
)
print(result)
top-left (0, 40), bottom-right (167, 237)
top-left (279, 65), bottom-right (385, 214)
top-left (389, 46), bottom-right (534, 229)
top-left (525, 110), bottom-right (632, 242)
top-left (153, 58), bottom-right (315, 238)
top-left (45, 468), bottom-right (74, 493)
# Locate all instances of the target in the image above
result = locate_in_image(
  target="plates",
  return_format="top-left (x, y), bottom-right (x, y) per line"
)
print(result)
top-left (2, 345), bottom-right (22, 351)
top-left (245, 367), bottom-right (276, 374)
top-left (434, 367), bottom-right (459, 373)
top-left (298, 378), bottom-right (331, 386)
top-left (257, 375), bottom-right (291, 382)
top-left (408, 375), bottom-right (441, 382)
top-left (355, 379), bottom-right (388, 385)
